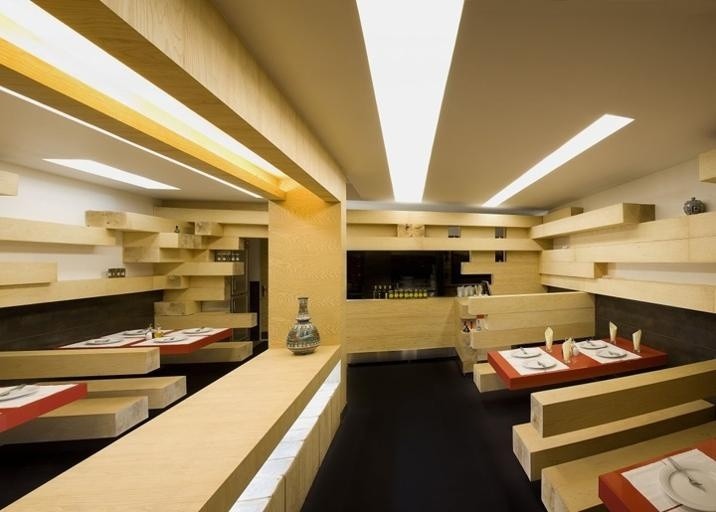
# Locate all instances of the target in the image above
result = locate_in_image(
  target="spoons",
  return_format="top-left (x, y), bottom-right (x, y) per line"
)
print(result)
top-left (666, 454), bottom-right (703, 486)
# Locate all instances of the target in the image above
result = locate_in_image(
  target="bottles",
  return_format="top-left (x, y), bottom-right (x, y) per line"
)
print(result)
top-left (175, 225), bottom-right (180, 233)
top-left (457, 284), bottom-right (474, 297)
top-left (216, 252), bottom-right (241, 263)
top-left (106, 268), bottom-right (126, 278)
top-left (373, 283), bottom-right (428, 298)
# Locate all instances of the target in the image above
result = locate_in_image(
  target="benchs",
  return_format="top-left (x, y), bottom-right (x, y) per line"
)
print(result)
top-left (154, 312), bottom-right (258, 363)
top-left (1, 349), bottom-right (188, 444)
top-left (468, 294), bottom-right (598, 394)
top-left (512, 356), bottom-right (716, 512)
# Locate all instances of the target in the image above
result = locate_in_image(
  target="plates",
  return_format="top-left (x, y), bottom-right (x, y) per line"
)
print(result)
top-left (579, 341), bottom-right (626, 358)
top-left (1, 388), bottom-right (40, 400)
top-left (87, 327), bottom-right (212, 345)
top-left (512, 349), bottom-right (556, 369)
top-left (659, 461), bottom-right (716, 511)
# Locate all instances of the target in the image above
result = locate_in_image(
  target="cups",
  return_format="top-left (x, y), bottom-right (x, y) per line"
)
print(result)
top-left (610, 329), bottom-right (617, 345)
top-left (562, 343), bottom-right (574, 365)
top-left (633, 336), bottom-right (642, 353)
top-left (545, 337), bottom-right (554, 354)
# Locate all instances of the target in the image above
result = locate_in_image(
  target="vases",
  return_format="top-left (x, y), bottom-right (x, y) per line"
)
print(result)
top-left (683, 198), bottom-right (706, 215)
top-left (286, 298), bottom-right (321, 355)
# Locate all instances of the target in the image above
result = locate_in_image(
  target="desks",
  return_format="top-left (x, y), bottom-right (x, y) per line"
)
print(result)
top-left (58, 325), bottom-right (231, 354)
top-left (0, 382), bottom-right (88, 439)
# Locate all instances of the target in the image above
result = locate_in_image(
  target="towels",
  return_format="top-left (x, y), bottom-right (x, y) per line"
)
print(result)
top-left (544, 326), bottom-right (553, 349)
top-left (562, 337), bottom-right (573, 360)
top-left (633, 330), bottom-right (643, 350)
top-left (609, 321), bottom-right (617, 342)
